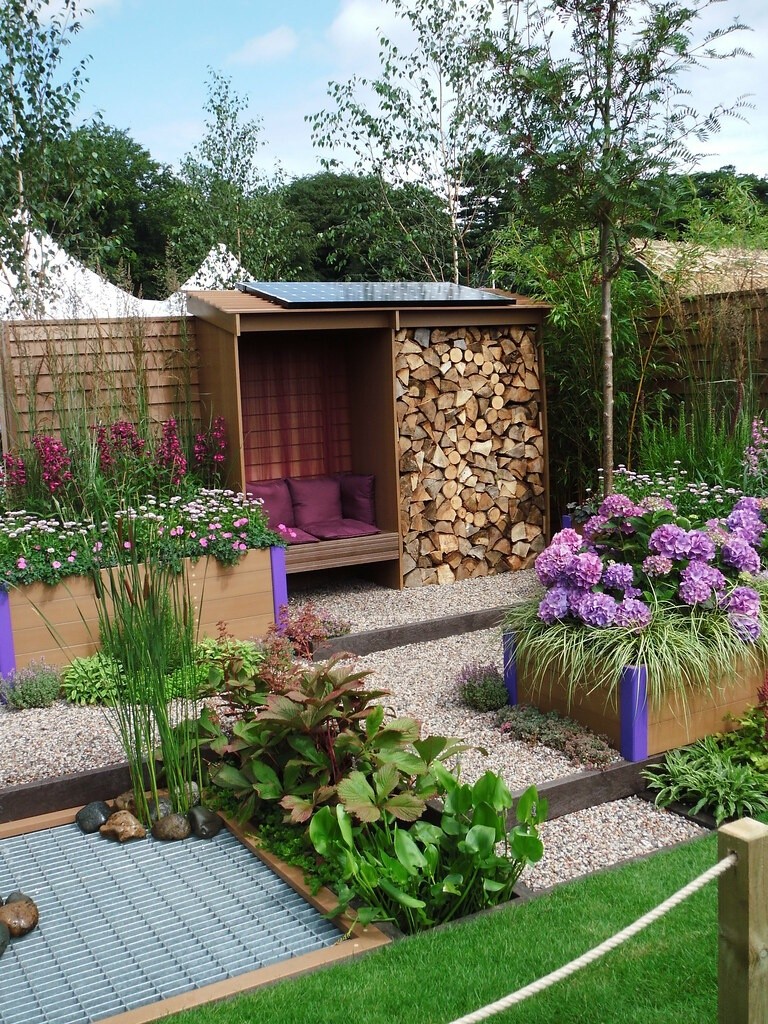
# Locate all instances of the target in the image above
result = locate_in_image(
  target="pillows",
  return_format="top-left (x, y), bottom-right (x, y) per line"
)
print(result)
top-left (339, 475), bottom-right (377, 524)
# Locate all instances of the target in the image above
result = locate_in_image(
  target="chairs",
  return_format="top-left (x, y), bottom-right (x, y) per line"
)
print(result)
top-left (285, 476), bottom-right (382, 540)
top-left (245, 479), bottom-right (320, 545)
